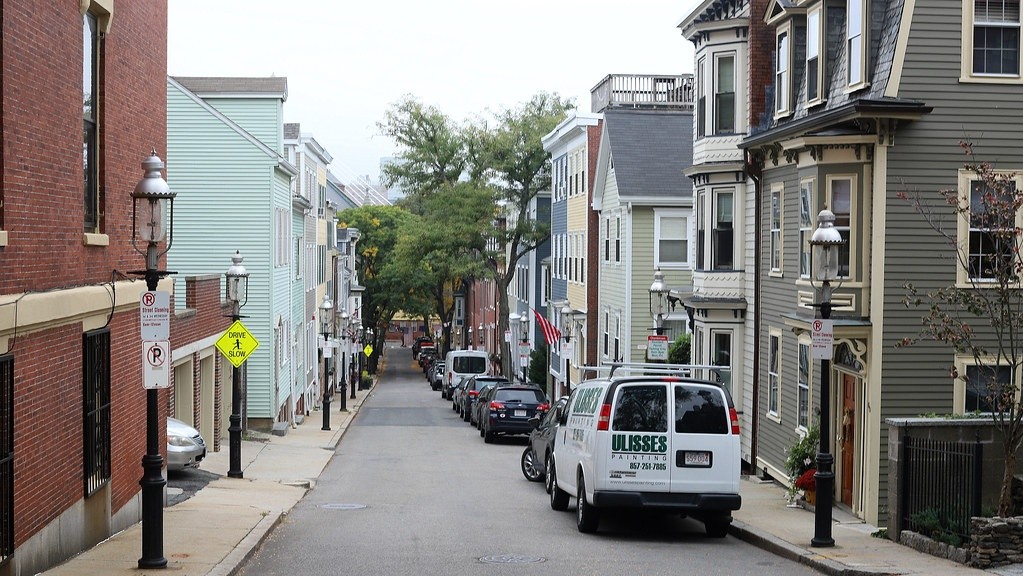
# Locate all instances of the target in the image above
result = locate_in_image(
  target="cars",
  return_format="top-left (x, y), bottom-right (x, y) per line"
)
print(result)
top-left (480, 384), bottom-right (552, 443)
top-left (414, 335), bottom-right (445, 391)
top-left (470, 383), bottom-right (495, 431)
top-left (165, 416), bottom-right (207, 474)
top-left (521, 394), bottom-right (568, 495)
top-left (452, 376), bottom-right (472, 413)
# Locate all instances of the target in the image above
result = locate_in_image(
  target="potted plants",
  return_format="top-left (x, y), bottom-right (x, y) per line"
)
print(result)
top-left (785, 422), bottom-right (820, 502)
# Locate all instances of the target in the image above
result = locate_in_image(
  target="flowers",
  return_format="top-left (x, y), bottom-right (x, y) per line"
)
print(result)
top-left (795, 469), bottom-right (820, 492)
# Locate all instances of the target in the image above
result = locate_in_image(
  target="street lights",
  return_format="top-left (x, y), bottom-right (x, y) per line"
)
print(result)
top-left (365, 327), bottom-right (374, 377)
top-left (128, 146), bottom-right (179, 570)
top-left (318, 292), bottom-right (335, 430)
top-left (645, 265), bottom-right (674, 375)
top-left (334, 306), bottom-right (351, 412)
top-left (560, 297), bottom-right (574, 396)
top-left (355, 322), bottom-right (364, 391)
top-left (222, 249), bottom-right (252, 479)
top-left (811, 202), bottom-right (874, 548)
top-left (520, 310), bottom-right (530, 383)
top-left (348, 314), bottom-right (360, 399)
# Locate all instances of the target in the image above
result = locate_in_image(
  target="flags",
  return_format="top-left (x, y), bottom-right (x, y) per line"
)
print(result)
top-left (533, 310), bottom-right (562, 345)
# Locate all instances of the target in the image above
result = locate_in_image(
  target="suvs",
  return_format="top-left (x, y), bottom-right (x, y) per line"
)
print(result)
top-left (459, 374), bottom-right (510, 422)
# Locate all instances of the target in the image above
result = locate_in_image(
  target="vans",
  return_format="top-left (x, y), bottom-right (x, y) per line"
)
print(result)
top-left (550, 361), bottom-right (743, 540)
top-left (439, 350), bottom-right (492, 401)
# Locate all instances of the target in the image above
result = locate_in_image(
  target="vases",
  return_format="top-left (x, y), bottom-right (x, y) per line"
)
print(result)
top-left (804, 490), bottom-right (818, 504)
